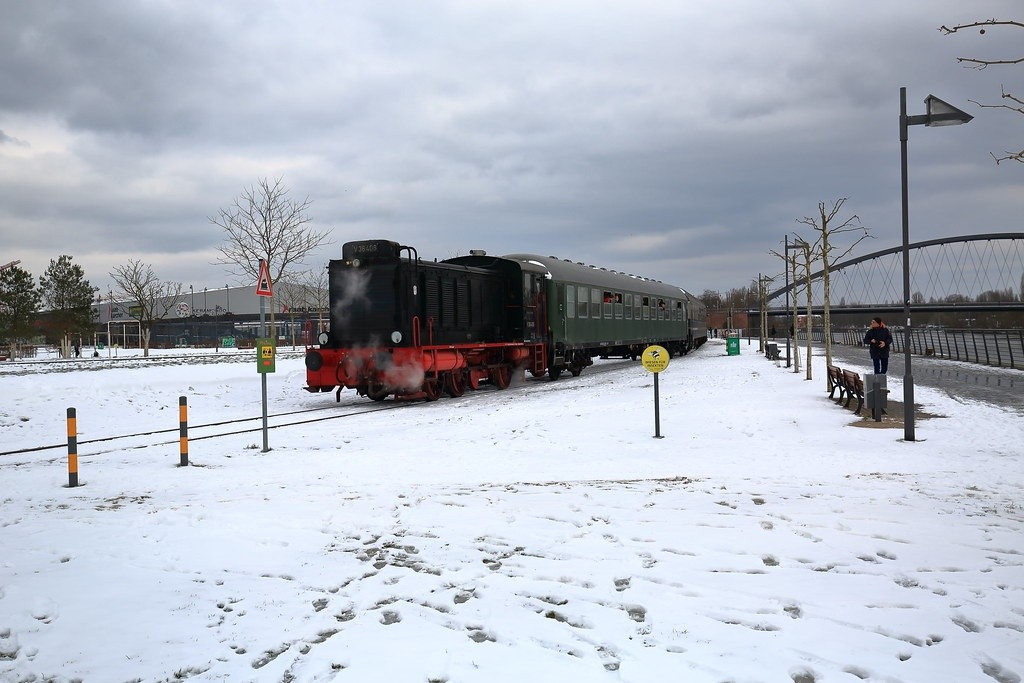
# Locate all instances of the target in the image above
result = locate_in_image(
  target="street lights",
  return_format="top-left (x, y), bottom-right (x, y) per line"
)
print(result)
top-left (202, 287), bottom-right (208, 316)
top-left (756, 272), bottom-right (775, 348)
top-left (189, 284), bottom-right (195, 318)
top-left (897, 86), bottom-right (975, 440)
top-left (784, 234), bottom-right (809, 368)
top-left (225, 282), bottom-right (230, 314)
top-left (113, 291), bottom-right (113, 327)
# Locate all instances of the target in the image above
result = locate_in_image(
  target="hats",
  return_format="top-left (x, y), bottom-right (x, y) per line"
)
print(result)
top-left (873, 318), bottom-right (881, 325)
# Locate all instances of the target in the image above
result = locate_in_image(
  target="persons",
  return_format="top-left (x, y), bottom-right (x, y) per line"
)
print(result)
top-left (604, 295), bottom-right (619, 302)
top-left (708, 326), bottom-right (717, 339)
top-left (770, 325), bottom-right (777, 335)
top-left (658, 299), bottom-right (666, 309)
top-left (74, 340), bottom-right (80, 358)
top-left (789, 323), bottom-right (794, 338)
top-left (863, 317), bottom-right (893, 374)
top-left (93, 347), bottom-right (99, 357)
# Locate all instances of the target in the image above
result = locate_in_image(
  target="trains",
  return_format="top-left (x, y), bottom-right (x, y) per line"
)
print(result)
top-left (304, 238), bottom-right (708, 403)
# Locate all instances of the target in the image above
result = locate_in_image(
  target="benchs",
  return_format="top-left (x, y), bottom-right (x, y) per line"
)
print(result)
top-left (765, 343), bottom-right (781, 360)
top-left (843, 369), bottom-right (890, 415)
top-left (827, 365), bottom-right (844, 405)
